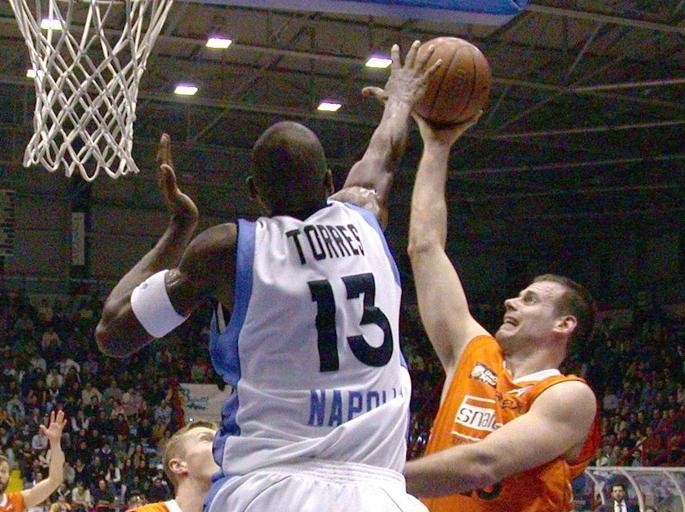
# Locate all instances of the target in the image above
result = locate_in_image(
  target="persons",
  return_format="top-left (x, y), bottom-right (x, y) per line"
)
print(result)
top-left (397, 98), bottom-right (605, 512)
top-left (91, 37), bottom-right (442, 510)
top-left (0, 256), bottom-right (683, 512)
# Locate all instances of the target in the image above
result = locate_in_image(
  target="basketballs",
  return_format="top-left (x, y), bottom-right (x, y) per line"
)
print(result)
top-left (412, 37), bottom-right (491, 123)
